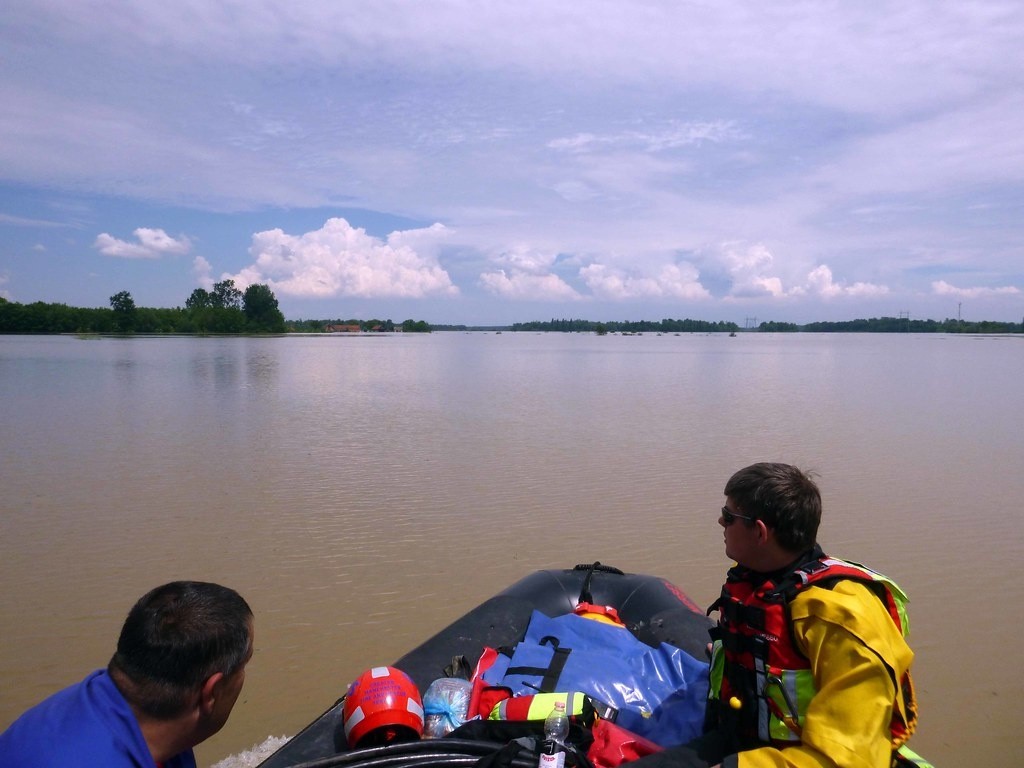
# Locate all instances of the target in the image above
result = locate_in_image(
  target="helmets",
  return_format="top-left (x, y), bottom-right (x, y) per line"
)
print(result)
top-left (342, 665), bottom-right (424, 751)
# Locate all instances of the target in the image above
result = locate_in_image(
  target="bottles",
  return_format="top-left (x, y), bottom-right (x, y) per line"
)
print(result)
top-left (538, 701), bottom-right (570, 768)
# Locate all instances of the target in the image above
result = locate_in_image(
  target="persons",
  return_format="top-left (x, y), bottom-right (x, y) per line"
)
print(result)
top-left (704, 462), bottom-right (919, 768)
top-left (0, 582), bottom-right (255, 768)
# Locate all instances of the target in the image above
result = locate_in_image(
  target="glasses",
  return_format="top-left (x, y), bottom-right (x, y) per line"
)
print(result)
top-left (722, 507), bottom-right (756, 526)
